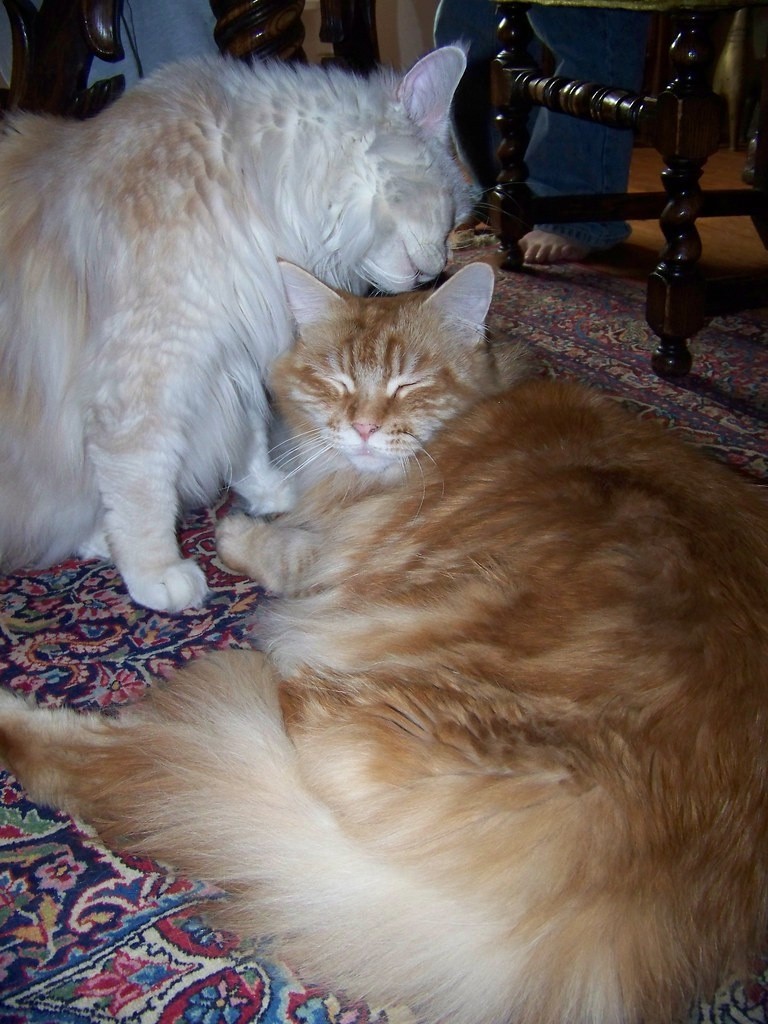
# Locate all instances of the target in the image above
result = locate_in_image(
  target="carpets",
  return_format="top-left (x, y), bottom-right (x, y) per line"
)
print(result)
top-left (0, 219), bottom-right (768, 1024)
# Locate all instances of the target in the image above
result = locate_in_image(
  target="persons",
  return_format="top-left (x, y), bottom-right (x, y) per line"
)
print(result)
top-left (84, 0), bottom-right (224, 93)
top-left (431, 0), bottom-right (652, 262)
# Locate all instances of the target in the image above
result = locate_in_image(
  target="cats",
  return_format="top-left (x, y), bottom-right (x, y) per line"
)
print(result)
top-left (0, 255), bottom-right (768, 1024)
top-left (0, 45), bottom-right (487, 614)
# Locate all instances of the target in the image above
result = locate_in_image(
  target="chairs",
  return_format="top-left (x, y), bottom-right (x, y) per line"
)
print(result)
top-left (486, 0), bottom-right (768, 379)
